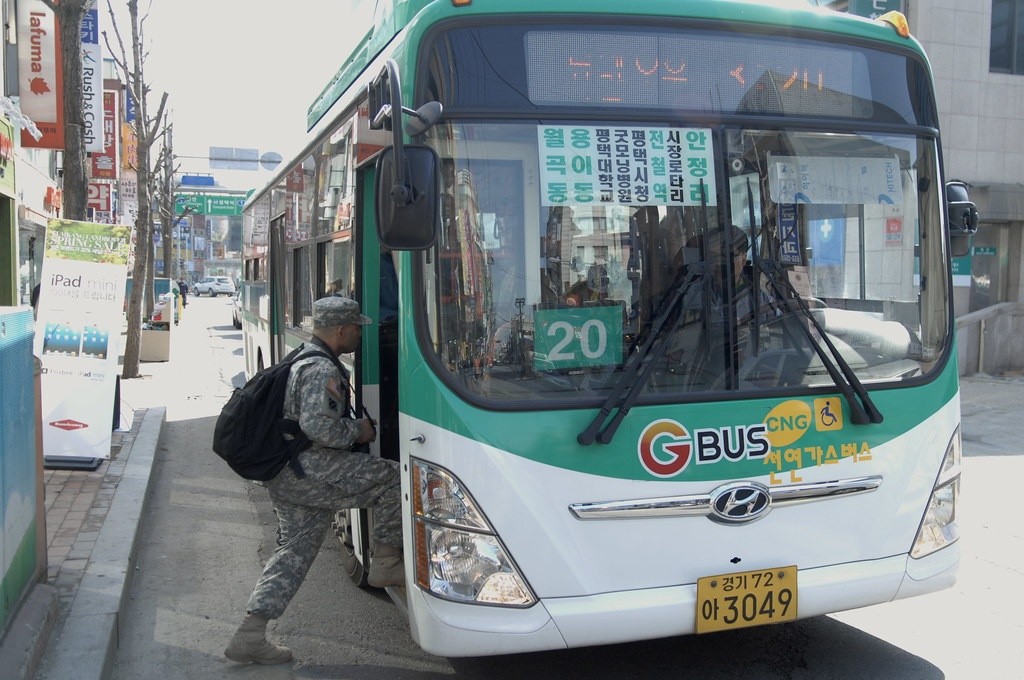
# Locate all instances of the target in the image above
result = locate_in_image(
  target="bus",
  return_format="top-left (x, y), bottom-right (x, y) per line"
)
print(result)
top-left (235, 1), bottom-right (982, 658)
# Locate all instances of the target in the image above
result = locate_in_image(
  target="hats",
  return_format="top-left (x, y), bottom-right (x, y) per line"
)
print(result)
top-left (313, 295), bottom-right (372, 325)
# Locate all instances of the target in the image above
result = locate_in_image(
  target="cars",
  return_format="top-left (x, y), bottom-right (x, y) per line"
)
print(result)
top-left (193, 276), bottom-right (235, 297)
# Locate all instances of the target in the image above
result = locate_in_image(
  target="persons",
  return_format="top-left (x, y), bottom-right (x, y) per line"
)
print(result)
top-left (177, 277), bottom-right (190, 308)
top-left (664, 222), bottom-right (795, 357)
top-left (221, 297), bottom-right (409, 666)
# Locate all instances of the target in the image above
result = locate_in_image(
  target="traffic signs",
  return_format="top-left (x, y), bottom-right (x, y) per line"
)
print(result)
top-left (175, 194), bottom-right (245, 218)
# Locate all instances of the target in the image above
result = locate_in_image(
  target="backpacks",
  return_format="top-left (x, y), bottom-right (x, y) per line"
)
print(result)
top-left (212, 359), bottom-right (313, 485)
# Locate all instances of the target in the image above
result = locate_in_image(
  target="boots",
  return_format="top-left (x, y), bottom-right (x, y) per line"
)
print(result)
top-left (223, 613), bottom-right (293, 663)
top-left (368, 542), bottom-right (406, 587)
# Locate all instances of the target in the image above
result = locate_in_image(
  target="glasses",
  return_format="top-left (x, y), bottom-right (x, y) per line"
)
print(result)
top-left (709, 251), bottom-right (743, 260)
